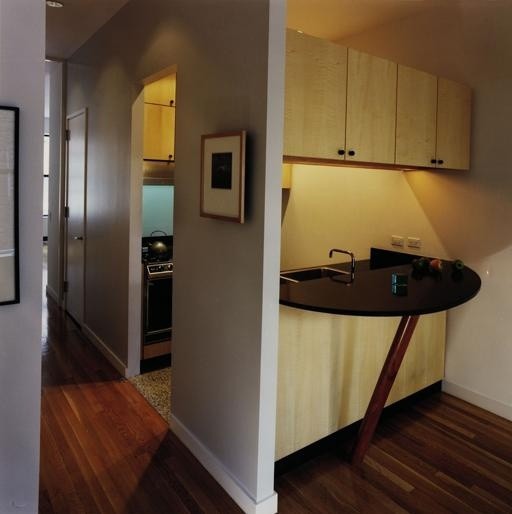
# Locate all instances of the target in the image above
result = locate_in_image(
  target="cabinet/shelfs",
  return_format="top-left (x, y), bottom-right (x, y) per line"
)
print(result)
top-left (143, 72), bottom-right (176, 185)
top-left (283, 26), bottom-right (470, 174)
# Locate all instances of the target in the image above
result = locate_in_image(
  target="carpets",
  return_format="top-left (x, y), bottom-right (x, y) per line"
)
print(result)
top-left (129, 366), bottom-right (172, 423)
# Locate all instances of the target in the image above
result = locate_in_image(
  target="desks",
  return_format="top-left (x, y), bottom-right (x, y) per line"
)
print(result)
top-left (280, 248), bottom-right (481, 471)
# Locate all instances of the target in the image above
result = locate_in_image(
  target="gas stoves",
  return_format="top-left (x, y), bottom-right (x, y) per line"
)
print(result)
top-left (142, 252), bottom-right (172, 278)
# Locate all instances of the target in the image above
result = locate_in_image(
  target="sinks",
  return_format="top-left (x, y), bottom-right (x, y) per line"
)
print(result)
top-left (280, 266), bottom-right (350, 282)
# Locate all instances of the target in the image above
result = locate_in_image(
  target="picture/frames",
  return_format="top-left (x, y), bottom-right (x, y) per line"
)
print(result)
top-left (200, 130), bottom-right (246, 225)
top-left (0, 105), bottom-right (20, 306)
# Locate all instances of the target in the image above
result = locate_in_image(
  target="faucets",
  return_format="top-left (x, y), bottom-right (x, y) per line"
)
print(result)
top-left (328, 273), bottom-right (355, 287)
top-left (329, 248), bottom-right (355, 273)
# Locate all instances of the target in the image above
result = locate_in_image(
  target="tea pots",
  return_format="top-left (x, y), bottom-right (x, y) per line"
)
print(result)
top-left (148, 231), bottom-right (172, 262)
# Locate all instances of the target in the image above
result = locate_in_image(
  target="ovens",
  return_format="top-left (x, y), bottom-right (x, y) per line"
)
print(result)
top-left (142, 278), bottom-right (172, 336)
